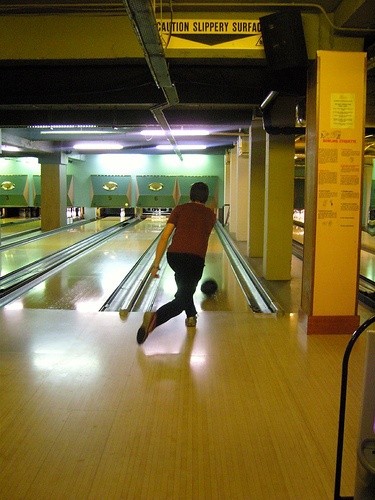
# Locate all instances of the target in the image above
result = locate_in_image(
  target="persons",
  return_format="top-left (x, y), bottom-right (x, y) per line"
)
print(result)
top-left (136, 182), bottom-right (216, 345)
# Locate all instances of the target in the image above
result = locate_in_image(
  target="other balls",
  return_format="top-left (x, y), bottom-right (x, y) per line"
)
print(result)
top-left (201, 279), bottom-right (217, 295)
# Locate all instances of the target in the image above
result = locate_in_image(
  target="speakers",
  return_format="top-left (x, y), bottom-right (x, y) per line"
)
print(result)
top-left (258, 8), bottom-right (309, 72)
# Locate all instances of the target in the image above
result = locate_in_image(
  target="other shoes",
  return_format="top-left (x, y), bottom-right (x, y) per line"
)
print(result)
top-left (137, 312), bottom-right (156, 345)
top-left (185, 316), bottom-right (197, 328)
